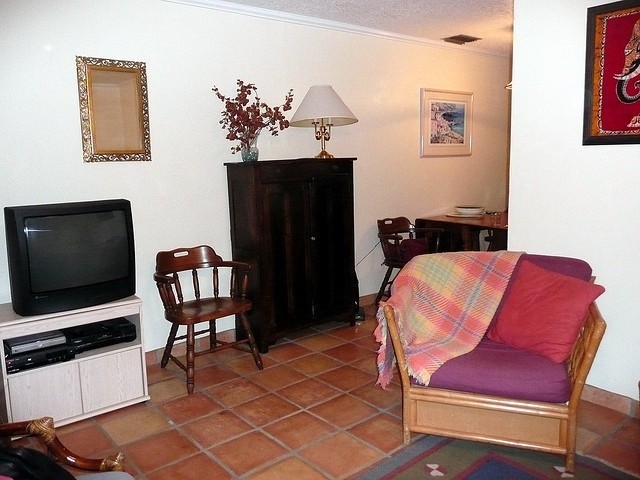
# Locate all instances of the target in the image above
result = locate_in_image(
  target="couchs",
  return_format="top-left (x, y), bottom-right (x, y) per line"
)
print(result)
top-left (383, 252), bottom-right (607, 473)
top-left (0, 416), bottom-right (134, 480)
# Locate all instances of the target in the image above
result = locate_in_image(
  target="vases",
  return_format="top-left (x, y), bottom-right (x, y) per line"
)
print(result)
top-left (241, 137), bottom-right (259, 161)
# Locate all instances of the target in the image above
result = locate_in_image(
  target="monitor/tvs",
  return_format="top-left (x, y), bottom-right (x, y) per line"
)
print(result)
top-left (4, 199), bottom-right (136, 316)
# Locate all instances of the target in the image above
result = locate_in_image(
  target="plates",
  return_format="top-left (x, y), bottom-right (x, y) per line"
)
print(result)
top-left (448, 212), bottom-right (488, 218)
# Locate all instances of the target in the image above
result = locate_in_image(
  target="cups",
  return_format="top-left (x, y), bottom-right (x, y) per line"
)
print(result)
top-left (489, 212), bottom-right (503, 226)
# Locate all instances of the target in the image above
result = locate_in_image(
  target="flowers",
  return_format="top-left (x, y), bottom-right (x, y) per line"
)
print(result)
top-left (211, 79), bottom-right (294, 155)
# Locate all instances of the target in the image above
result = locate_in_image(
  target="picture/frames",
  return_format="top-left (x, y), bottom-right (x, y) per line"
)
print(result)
top-left (76, 55), bottom-right (151, 162)
top-left (582, 0), bottom-right (640, 145)
top-left (420, 88), bottom-right (474, 157)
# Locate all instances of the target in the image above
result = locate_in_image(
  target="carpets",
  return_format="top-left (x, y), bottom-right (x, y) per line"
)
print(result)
top-left (346, 434), bottom-right (640, 480)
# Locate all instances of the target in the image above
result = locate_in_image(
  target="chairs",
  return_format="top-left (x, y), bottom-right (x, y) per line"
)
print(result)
top-left (153, 245), bottom-right (263, 394)
top-left (374, 217), bottom-right (444, 305)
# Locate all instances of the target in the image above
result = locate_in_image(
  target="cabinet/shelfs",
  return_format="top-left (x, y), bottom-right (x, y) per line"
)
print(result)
top-left (223, 157), bottom-right (359, 354)
top-left (0, 295), bottom-right (150, 442)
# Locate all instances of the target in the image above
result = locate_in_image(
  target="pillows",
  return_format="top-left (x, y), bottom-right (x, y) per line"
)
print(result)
top-left (487, 260), bottom-right (605, 364)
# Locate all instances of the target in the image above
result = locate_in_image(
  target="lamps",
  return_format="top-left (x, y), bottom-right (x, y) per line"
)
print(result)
top-left (289, 85), bottom-right (359, 159)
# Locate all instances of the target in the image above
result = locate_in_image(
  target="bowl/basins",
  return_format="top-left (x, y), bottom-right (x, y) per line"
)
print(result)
top-left (456, 206), bottom-right (484, 216)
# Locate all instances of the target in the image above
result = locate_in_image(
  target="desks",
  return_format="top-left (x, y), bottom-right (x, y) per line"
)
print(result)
top-left (414, 211), bottom-right (507, 251)
